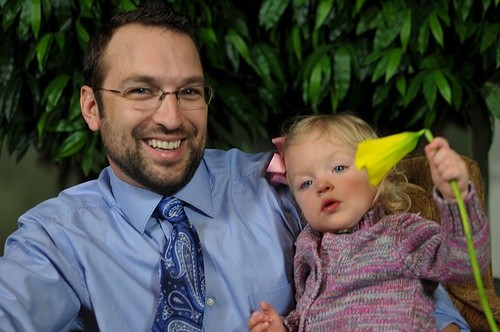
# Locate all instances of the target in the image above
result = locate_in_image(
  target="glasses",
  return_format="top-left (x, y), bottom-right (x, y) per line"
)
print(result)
top-left (94, 84), bottom-right (214, 110)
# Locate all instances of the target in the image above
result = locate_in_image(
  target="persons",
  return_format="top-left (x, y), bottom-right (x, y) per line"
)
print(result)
top-left (246, 114), bottom-right (492, 332)
top-left (0, 8), bottom-right (470, 332)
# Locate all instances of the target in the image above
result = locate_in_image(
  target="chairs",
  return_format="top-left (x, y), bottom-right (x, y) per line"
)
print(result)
top-left (396, 155), bottom-right (500, 332)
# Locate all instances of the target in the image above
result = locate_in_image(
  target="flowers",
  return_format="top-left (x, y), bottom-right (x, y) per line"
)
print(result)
top-left (356, 130), bottom-right (500, 332)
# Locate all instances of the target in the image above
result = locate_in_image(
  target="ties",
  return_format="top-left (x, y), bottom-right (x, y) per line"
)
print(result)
top-left (148, 195), bottom-right (207, 332)
